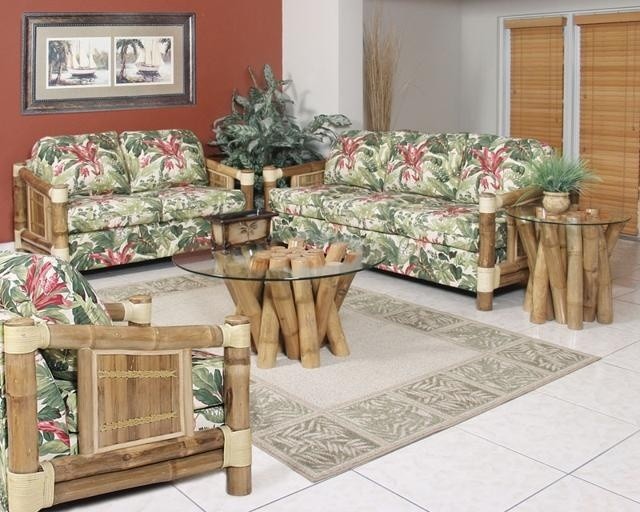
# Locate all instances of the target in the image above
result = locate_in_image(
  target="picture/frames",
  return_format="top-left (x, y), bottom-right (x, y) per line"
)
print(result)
top-left (19, 12), bottom-right (195, 115)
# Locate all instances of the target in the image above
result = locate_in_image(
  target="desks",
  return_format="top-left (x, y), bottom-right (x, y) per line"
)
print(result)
top-left (504, 204), bottom-right (634, 330)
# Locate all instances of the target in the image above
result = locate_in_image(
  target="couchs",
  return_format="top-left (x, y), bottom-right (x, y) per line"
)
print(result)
top-left (13, 128), bottom-right (255, 271)
top-left (262, 130), bottom-right (582, 311)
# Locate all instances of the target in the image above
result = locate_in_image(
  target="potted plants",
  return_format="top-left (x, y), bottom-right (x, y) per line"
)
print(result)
top-left (511, 147), bottom-right (603, 213)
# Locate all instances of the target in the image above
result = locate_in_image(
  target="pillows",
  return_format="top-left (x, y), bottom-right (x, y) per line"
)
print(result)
top-left (324, 128), bottom-right (383, 192)
top-left (455, 133), bottom-right (555, 206)
top-left (0, 246), bottom-right (114, 382)
top-left (382, 129), bottom-right (468, 199)
top-left (119, 128), bottom-right (211, 191)
top-left (31, 129), bottom-right (131, 194)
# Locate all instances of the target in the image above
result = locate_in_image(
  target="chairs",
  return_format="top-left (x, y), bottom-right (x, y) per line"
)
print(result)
top-left (0, 296), bottom-right (253, 512)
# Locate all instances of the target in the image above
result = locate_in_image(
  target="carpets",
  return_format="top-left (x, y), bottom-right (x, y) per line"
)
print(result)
top-left (97, 268), bottom-right (604, 483)
